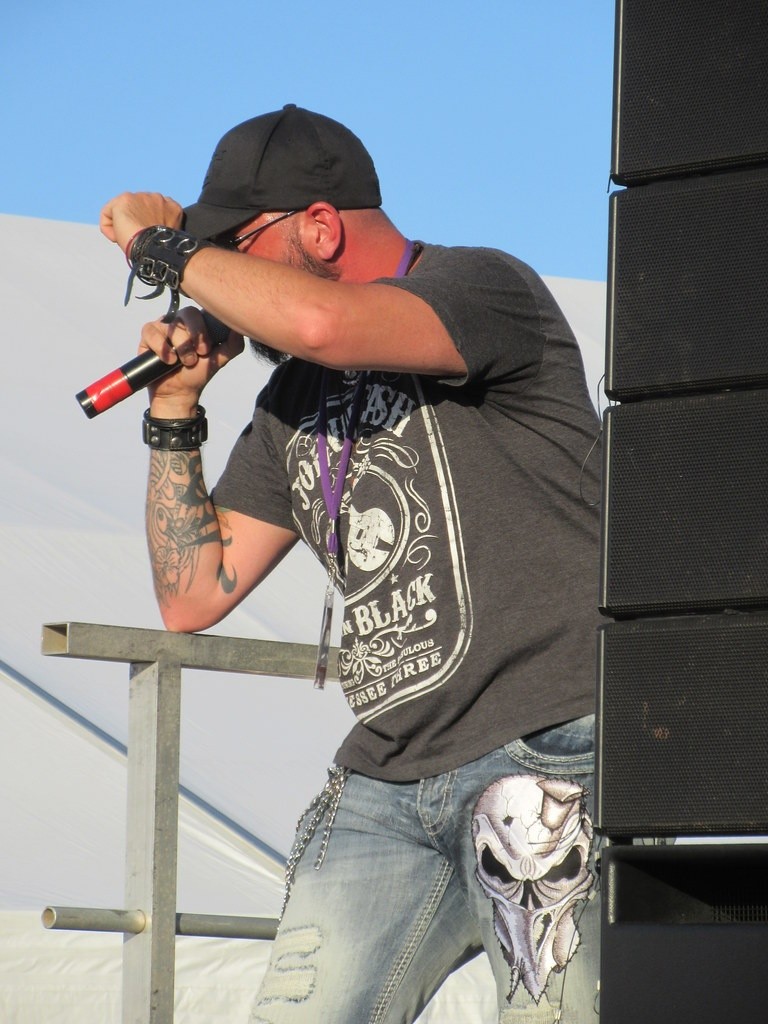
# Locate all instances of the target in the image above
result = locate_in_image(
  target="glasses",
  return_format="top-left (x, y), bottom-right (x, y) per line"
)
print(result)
top-left (216, 211), bottom-right (296, 251)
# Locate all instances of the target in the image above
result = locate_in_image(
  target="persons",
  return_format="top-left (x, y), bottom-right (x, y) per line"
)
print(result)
top-left (98, 102), bottom-right (604, 1024)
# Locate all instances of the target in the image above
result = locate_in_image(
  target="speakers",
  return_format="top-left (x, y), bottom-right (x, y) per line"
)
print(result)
top-left (600, 0), bottom-right (768, 1024)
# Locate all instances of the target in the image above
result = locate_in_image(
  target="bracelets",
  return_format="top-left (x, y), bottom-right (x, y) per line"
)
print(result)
top-left (124, 224), bottom-right (217, 325)
top-left (141, 404), bottom-right (209, 450)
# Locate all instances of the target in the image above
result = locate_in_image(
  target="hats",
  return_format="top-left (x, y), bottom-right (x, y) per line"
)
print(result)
top-left (180, 104), bottom-right (381, 241)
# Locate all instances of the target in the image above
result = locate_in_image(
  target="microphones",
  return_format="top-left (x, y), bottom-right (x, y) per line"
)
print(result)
top-left (75, 307), bottom-right (229, 419)
